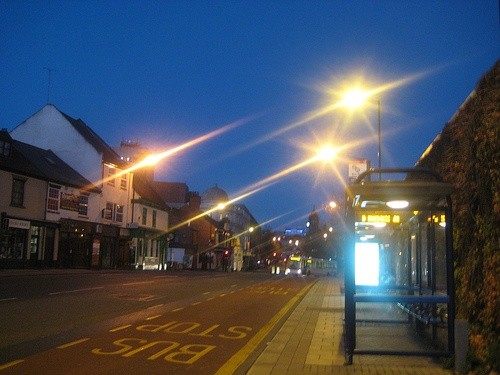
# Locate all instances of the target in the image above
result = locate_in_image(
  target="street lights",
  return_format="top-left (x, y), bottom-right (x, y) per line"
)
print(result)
top-left (340, 82), bottom-right (384, 182)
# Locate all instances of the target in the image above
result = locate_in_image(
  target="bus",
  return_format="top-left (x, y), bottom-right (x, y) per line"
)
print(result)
top-left (284, 255), bottom-right (338, 279)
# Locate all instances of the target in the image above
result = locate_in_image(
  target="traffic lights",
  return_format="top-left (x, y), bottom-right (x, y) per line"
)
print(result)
top-left (223, 249), bottom-right (230, 265)
top-left (272, 252), bottom-right (278, 264)
